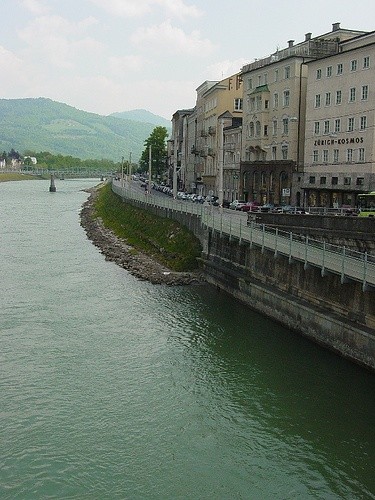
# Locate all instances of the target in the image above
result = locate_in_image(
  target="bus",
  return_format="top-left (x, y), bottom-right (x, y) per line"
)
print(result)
top-left (355, 190), bottom-right (375, 218)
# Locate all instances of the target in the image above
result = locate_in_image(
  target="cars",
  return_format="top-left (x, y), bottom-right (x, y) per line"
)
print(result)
top-left (132, 175), bottom-right (308, 214)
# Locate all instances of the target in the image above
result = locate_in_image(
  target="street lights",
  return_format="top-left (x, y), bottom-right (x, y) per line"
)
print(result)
top-left (129, 152), bottom-right (132, 190)
top-left (121, 156), bottom-right (125, 187)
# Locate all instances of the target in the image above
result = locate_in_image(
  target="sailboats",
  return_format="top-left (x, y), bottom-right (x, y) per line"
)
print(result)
top-left (60, 174), bottom-right (64, 180)
top-left (50, 174), bottom-right (56, 192)
top-left (101, 176), bottom-right (104, 181)
